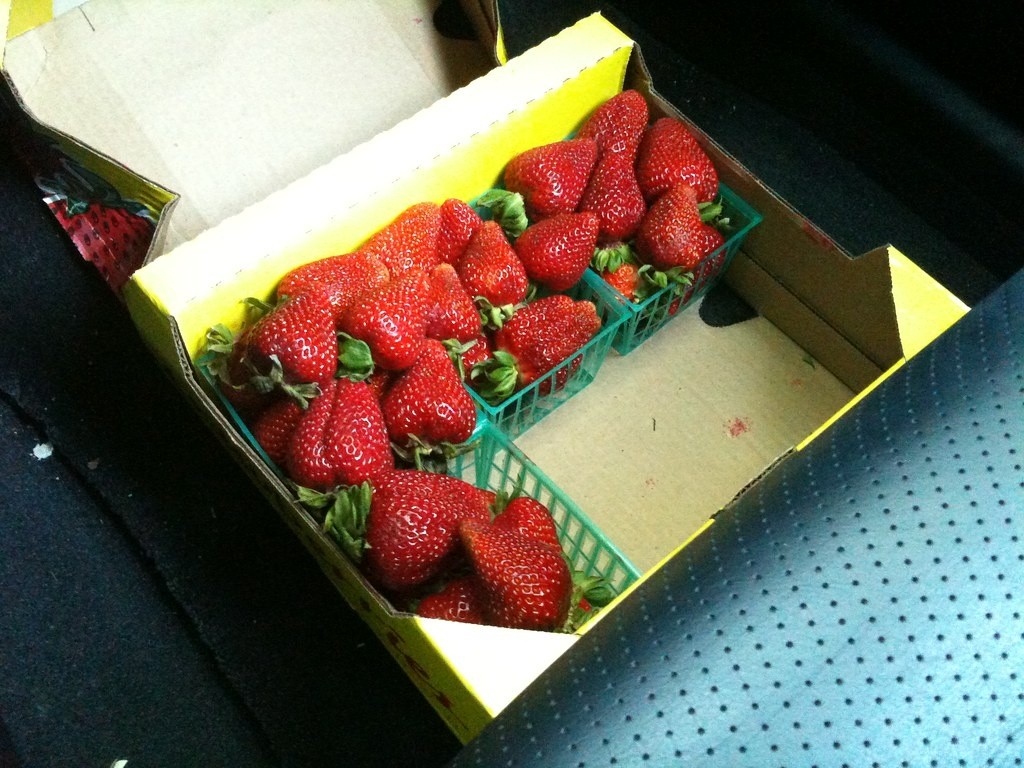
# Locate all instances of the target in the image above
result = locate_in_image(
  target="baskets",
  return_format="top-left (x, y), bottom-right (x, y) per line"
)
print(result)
top-left (193, 128), bottom-right (767, 595)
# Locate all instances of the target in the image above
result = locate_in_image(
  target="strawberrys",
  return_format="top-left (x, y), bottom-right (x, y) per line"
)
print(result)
top-left (211, 91), bottom-right (727, 633)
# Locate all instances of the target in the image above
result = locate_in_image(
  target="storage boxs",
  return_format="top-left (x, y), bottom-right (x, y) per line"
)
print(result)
top-left (0, 0), bottom-right (974, 742)
